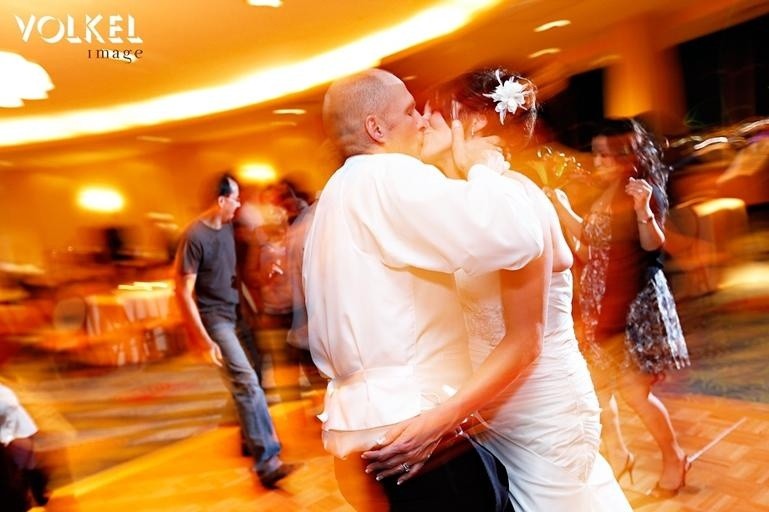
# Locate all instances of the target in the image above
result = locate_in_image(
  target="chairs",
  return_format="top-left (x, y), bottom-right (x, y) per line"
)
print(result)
top-left (98, 304), bottom-right (159, 369)
top-left (48, 297), bottom-right (86, 378)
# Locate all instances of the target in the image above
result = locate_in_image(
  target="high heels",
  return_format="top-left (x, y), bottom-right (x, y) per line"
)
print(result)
top-left (617, 452), bottom-right (635, 485)
top-left (650, 454), bottom-right (692, 499)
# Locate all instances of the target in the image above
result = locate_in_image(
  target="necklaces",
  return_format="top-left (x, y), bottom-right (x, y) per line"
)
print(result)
top-left (598, 182), bottom-right (618, 207)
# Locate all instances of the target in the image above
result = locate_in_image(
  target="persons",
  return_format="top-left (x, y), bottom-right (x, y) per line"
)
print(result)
top-left (541, 115), bottom-right (695, 503)
top-left (300, 66), bottom-right (544, 510)
top-left (0, 380), bottom-right (54, 510)
top-left (174, 170), bottom-right (332, 487)
top-left (358, 68), bottom-right (636, 511)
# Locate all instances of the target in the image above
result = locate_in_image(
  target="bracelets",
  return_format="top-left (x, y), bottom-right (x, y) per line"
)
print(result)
top-left (638, 211), bottom-right (655, 225)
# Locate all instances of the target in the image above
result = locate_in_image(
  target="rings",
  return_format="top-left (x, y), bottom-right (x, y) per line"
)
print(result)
top-left (400, 463), bottom-right (410, 472)
top-left (637, 188), bottom-right (643, 194)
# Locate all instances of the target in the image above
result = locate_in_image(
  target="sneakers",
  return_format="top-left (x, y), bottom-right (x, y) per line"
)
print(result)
top-left (260, 461), bottom-right (302, 485)
